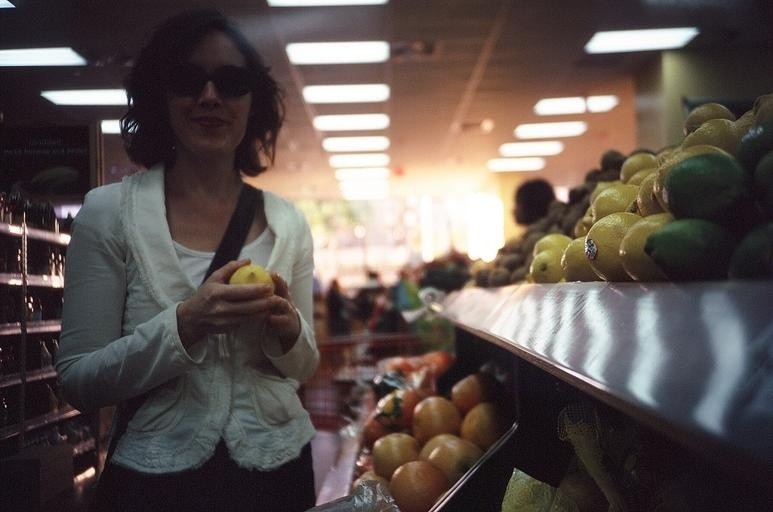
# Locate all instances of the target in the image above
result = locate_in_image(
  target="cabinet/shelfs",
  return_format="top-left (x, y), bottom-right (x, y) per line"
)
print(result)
top-left (350, 279), bottom-right (771, 511)
top-left (0, 209), bottom-right (104, 510)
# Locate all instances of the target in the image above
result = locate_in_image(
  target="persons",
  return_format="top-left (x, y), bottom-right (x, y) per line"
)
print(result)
top-left (513, 180), bottom-right (555, 226)
top-left (326, 280), bottom-right (349, 336)
top-left (51, 12), bottom-right (320, 508)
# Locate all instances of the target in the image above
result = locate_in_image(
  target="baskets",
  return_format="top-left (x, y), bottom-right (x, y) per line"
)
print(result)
top-left (304, 331), bottom-right (421, 434)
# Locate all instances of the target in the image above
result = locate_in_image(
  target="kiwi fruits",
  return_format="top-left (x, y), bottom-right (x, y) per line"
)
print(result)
top-left (475, 147), bottom-right (655, 285)
top-left (559, 473), bottom-right (611, 512)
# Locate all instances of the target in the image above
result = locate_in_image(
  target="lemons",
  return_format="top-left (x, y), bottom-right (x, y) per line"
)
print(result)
top-left (229, 265), bottom-right (275, 297)
top-left (530, 94), bottom-right (773, 286)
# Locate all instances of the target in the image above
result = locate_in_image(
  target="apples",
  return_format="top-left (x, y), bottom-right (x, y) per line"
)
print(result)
top-left (359, 351), bottom-right (511, 511)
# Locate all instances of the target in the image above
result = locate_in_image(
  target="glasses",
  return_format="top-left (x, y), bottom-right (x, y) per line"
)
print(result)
top-left (171, 65), bottom-right (255, 99)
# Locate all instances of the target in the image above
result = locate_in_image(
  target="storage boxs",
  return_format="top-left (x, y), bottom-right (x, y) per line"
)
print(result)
top-left (0, 441), bottom-right (77, 507)
